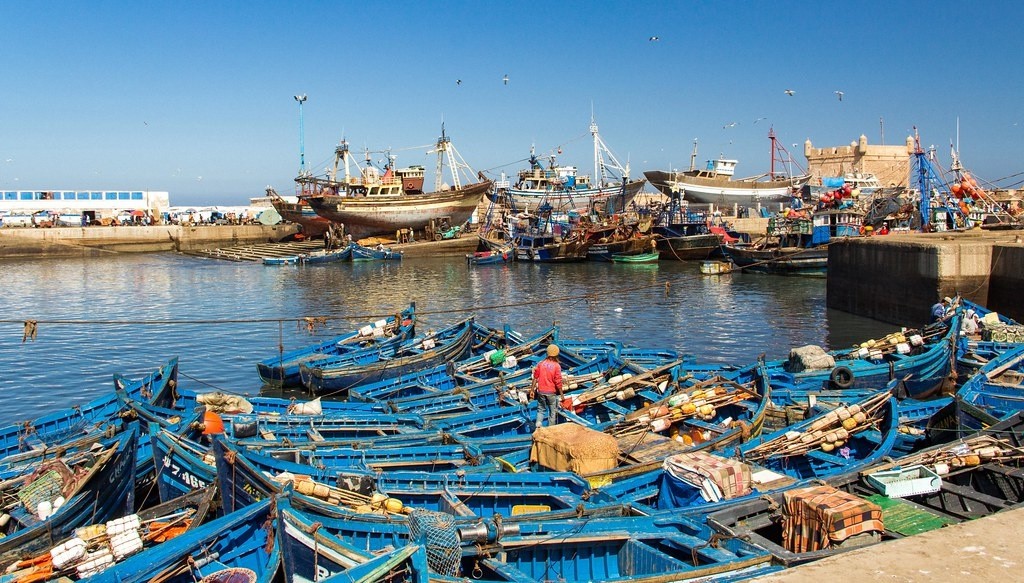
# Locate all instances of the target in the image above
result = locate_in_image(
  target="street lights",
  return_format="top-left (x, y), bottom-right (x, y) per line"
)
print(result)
top-left (294, 93), bottom-right (308, 173)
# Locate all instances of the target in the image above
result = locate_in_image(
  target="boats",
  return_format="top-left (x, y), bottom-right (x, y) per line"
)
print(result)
top-left (264, 98), bottom-right (1023, 278)
top-left (1, 301), bottom-right (1024, 583)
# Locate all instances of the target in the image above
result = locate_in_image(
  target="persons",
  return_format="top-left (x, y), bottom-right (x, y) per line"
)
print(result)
top-left (530, 344), bottom-right (565, 428)
top-left (395, 228), bottom-right (414, 244)
top-left (325, 222), bottom-right (353, 248)
top-left (962, 309), bottom-right (985, 341)
top-left (933, 296), bottom-right (952, 329)
top-left (440, 219), bottom-right (449, 231)
top-left (169, 213), bottom-right (244, 225)
top-left (32, 215), bottom-right (154, 226)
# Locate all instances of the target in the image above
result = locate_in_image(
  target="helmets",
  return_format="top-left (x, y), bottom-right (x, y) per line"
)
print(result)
top-left (546, 345), bottom-right (559, 357)
top-left (945, 297), bottom-right (951, 303)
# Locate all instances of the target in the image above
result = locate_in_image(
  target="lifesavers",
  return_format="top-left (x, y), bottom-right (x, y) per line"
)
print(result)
top-left (408, 183), bottom-right (414, 189)
top-left (860, 226), bottom-right (865, 235)
top-left (831, 366), bottom-right (855, 388)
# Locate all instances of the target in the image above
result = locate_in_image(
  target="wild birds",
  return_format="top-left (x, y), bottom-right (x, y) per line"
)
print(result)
top-left (502, 74), bottom-right (509, 85)
top-left (649, 37), bottom-right (660, 41)
top-left (834, 91), bottom-right (845, 102)
top-left (753, 118), bottom-right (767, 124)
top-left (456, 80), bottom-right (462, 85)
top-left (784, 90), bottom-right (795, 96)
top-left (724, 122), bottom-right (741, 129)
